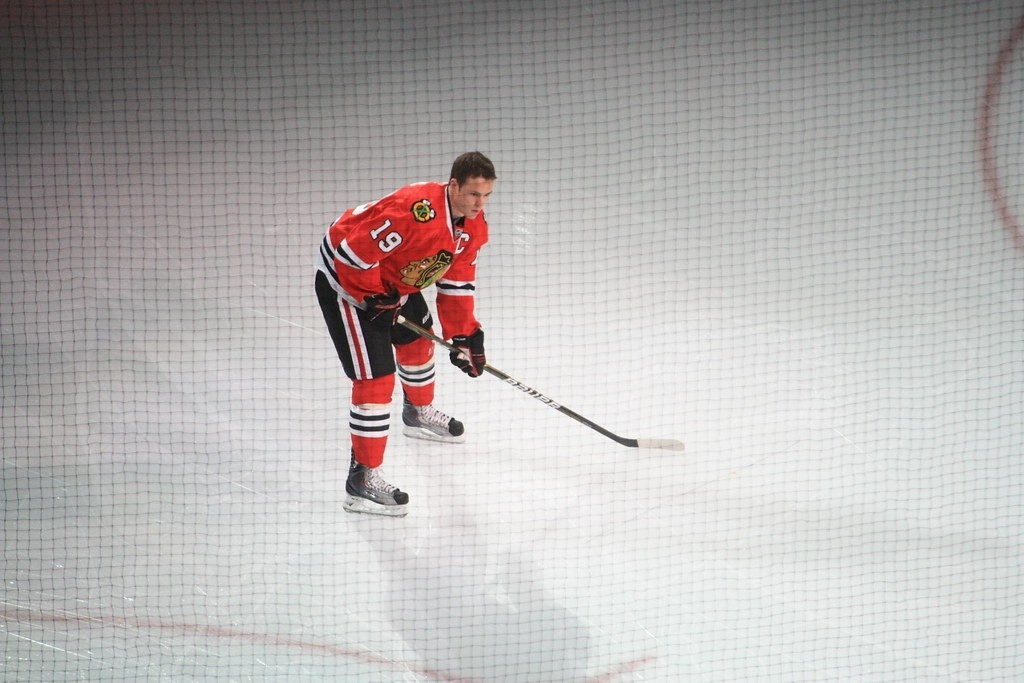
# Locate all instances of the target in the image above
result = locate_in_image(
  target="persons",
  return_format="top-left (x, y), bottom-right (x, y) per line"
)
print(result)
top-left (315, 150), bottom-right (498, 517)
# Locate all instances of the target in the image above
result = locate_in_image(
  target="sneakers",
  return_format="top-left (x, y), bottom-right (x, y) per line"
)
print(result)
top-left (344, 456), bottom-right (410, 518)
top-left (401, 397), bottom-right (467, 445)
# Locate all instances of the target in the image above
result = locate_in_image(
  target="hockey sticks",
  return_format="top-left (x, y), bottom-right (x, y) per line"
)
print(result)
top-left (395, 313), bottom-right (687, 453)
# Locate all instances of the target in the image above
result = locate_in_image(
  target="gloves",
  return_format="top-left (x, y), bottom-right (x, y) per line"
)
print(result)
top-left (366, 290), bottom-right (401, 329)
top-left (448, 330), bottom-right (486, 379)
